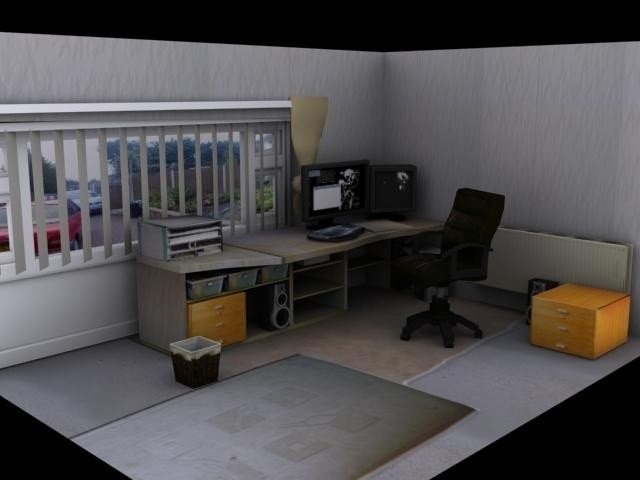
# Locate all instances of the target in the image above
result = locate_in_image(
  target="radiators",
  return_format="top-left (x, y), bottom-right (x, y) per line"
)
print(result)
top-left (478, 227), bottom-right (635, 301)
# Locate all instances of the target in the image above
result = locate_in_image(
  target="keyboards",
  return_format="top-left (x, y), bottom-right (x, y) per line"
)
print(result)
top-left (308, 225), bottom-right (366, 242)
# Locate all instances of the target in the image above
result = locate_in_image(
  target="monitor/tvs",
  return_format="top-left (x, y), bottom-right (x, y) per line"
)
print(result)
top-left (370, 165), bottom-right (417, 222)
top-left (301, 159), bottom-right (370, 230)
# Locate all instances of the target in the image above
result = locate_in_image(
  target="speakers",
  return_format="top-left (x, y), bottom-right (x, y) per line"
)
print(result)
top-left (527, 279), bottom-right (558, 325)
top-left (260, 281), bottom-right (290, 331)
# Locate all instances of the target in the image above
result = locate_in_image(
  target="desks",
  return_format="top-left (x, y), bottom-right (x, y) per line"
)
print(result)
top-left (226, 211), bottom-right (448, 331)
top-left (133, 244), bottom-right (284, 355)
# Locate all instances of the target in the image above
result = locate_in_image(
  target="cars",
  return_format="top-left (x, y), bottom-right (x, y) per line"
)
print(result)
top-left (1, 188), bottom-right (103, 256)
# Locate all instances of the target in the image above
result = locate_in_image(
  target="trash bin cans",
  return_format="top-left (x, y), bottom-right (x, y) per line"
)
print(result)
top-left (169, 336), bottom-right (221, 388)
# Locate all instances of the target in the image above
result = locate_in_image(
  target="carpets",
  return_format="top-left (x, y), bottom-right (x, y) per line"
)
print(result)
top-left (68, 354), bottom-right (478, 480)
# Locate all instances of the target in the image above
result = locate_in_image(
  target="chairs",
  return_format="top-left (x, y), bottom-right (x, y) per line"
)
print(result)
top-left (388, 185), bottom-right (506, 348)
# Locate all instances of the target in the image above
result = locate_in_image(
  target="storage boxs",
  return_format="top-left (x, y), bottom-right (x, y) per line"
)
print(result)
top-left (529, 282), bottom-right (633, 361)
top-left (185, 263), bottom-right (290, 348)
